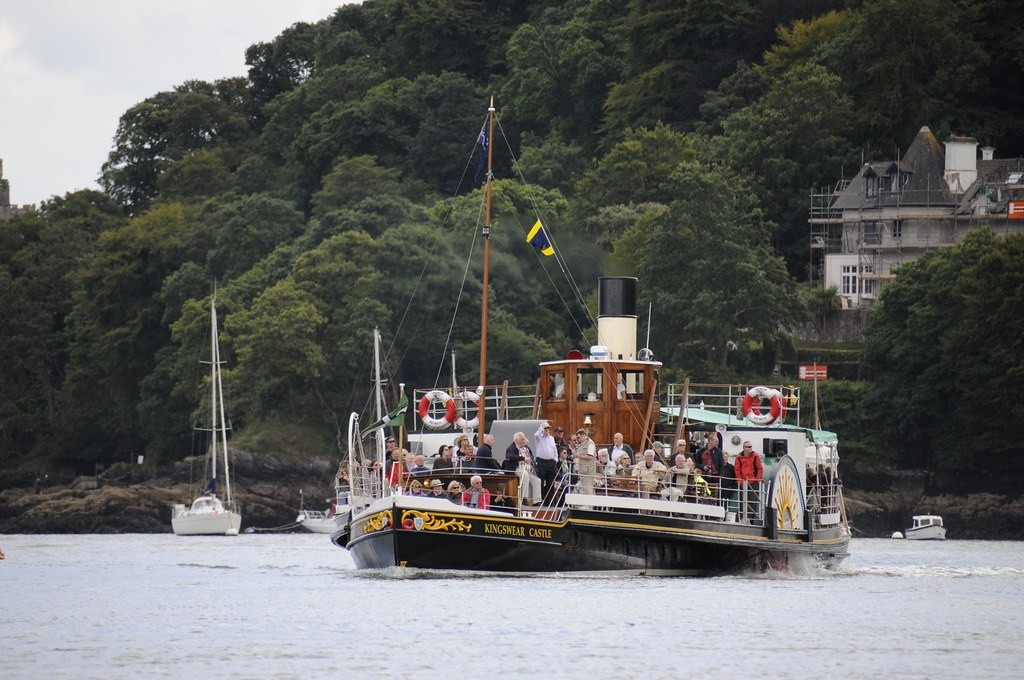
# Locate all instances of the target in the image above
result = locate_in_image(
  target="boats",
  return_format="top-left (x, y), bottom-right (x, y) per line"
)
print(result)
top-left (905, 512), bottom-right (949, 540)
top-left (296, 488), bottom-right (333, 535)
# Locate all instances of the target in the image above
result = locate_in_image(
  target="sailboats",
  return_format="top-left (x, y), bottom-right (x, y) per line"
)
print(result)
top-left (170, 297), bottom-right (246, 538)
top-left (329, 93), bottom-right (855, 579)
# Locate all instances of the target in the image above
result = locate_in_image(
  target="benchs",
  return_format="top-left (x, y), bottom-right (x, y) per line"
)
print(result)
top-left (597, 472), bottom-right (719, 522)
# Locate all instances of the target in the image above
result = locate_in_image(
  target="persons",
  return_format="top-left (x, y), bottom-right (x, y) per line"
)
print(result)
top-left (735, 441), bottom-right (763, 524)
top-left (553, 427), bottom-right (734, 520)
top-left (506, 432), bottom-right (543, 506)
top-left (617, 376), bottom-right (626, 400)
top-left (336, 459), bottom-right (384, 512)
top-left (406, 476), bottom-right (517, 517)
top-left (806, 462), bottom-right (843, 529)
top-left (534, 421), bottom-right (559, 506)
top-left (380, 434), bottom-right (474, 491)
top-left (472, 435), bottom-right (506, 475)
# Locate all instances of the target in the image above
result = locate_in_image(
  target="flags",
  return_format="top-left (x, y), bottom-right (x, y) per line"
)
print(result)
top-left (359, 391), bottom-right (409, 442)
top-left (475, 119), bottom-right (490, 190)
top-left (526, 219), bottom-right (555, 257)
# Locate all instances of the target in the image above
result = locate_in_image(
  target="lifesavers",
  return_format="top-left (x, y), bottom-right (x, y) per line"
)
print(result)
top-left (751, 388), bottom-right (788, 428)
top-left (742, 386), bottom-right (780, 424)
top-left (418, 390), bottom-right (456, 431)
top-left (453, 391), bottom-right (480, 429)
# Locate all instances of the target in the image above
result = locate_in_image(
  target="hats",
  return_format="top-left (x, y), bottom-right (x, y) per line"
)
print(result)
top-left (644, 450), bottom-right (655, 457)
top-left (554, 427), bottom-right (564, 432)
top-left (430, 479), bottom-right (444, 488)
top-left (540, 422), bottom-right (551, 429)
top-left (497, 483), bottom-right (505, 490)
top-left (653, 442), bottom-right (663, 447)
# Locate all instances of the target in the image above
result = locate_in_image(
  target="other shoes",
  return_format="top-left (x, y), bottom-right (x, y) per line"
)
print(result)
top-left (641, 510), bottom-right (651, 515)
top-left (533, 502), bottom-right (541, 506)
top-left (522, 499), bottom-right (528, 506)
top-left (672, 512), bottom-right (679, 517)
top-left (660, 512), bottom-right (669, 516)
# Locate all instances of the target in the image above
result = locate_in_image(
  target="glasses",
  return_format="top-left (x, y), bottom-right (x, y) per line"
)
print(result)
top-left (820, 469), bottom-right (823, 471)
top-left (452, 487), bottom-right (459, 489)
top-left (375, 464), bottom-right (377, 466)
top-left (622, 458), bottom-right (629, 460)
top-left (679, 444), bottom-right (685, 447)
top-left (476, 482), bottom-right (482, 484)
top-left (389, 440), bottom-right (395, 443)
top-left (415, 486), bottom-right (421, 489)
top-left (745, 446), bottom-right (752, 448)
top-left (463, 439), bottom-right (468, 441)
